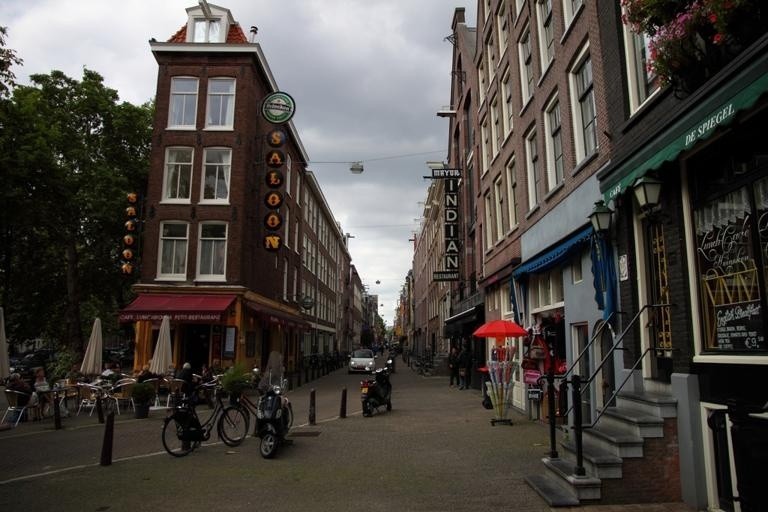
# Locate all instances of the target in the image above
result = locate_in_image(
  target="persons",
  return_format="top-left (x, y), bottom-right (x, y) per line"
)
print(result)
top-left (137, 364), bottom-right (156, 382)
top-left (9, 372), bottom-right (44, 421)
top-left (180, 362), bottom-right (195, 389)
top-left (64, 364), bottom-right (82, 382)
top-left (458, 343), bottom-right (474, 390)
top-left (202, 364), bottom-right (214, 383)
top-left (33, 365), bottom-right (54, 417)
top-left (448, 347), bottom-right (461, 388)
top-left (368, 338), bottom-right (391, 358)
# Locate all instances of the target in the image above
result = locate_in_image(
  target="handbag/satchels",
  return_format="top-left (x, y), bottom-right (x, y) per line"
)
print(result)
top-left (520, 331), bottom-right (547, 402)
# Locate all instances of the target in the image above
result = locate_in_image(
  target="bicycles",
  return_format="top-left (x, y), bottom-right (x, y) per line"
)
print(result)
top-left (160, 374), bottom-right (252, 458)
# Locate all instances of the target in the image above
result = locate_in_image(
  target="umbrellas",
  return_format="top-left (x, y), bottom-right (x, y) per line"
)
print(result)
top-left (147, 314), bottom-right (174, 379)
top-left (473, 318), bottom-right (530, 340)
top-left (1, 306), bottom-right (12, 386)
top-left (483, 344), bottom-right (519, 420)
top-left (79, 317), bottom-right (104, 380)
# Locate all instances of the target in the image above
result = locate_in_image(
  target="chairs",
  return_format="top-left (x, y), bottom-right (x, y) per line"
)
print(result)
top-left (2, 361), bottom-right (224, 427)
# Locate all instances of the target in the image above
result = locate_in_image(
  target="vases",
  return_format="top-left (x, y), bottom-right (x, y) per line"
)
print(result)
top-left (672, 62), bottom-right (711, 91)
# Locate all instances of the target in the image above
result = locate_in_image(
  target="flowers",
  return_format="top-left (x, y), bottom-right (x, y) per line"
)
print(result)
top-left (622, 0), bottom-right (760, 83)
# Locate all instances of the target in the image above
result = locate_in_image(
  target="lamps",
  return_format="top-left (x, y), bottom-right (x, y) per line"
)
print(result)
top-left (631, 172), bottom-right (666, 217)
top-left (587, 200), bottom-right (619, 237)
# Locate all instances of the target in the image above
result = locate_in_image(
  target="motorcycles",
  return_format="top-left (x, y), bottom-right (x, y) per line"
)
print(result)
top-left (359, 359), bottom-right (394, 418)
top-left (383, 357), bottom-right (395, 374)
top-left (252, 365), bottom-right (293, 458)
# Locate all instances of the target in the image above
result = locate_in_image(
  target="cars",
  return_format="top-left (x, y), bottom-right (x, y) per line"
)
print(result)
top-left (9, 349), bottom-right (54, 376)
top-left (347, 348), bottom-right (379, 375)
